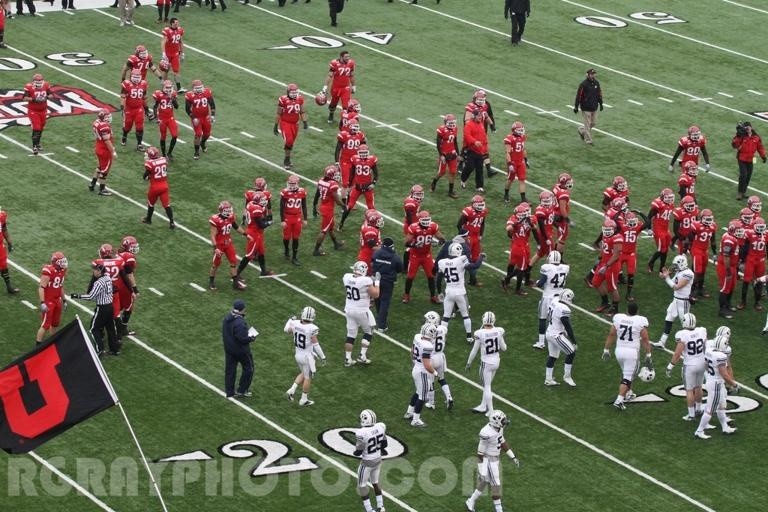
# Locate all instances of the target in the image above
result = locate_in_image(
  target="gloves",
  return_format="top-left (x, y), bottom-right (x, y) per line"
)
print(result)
top-left (70, 293), bottom-right (80, 300)
top-left (40, 303), bottom-right (50, 313)
top-left (63, 301), bottom-right (68, 313)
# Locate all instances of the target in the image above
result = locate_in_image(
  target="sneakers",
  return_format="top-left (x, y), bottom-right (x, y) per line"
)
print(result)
top-left (91, 326), bottom-right (137, 358)
top-left (5, 0), bottom-right (338, 28)
top-left (7, 286), bottom-right (21, 296)
top-left (199, 272), bottom-right (767, 512)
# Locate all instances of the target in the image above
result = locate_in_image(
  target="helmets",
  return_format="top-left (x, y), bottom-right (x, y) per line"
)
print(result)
top-left (0, 44), bottom-right (768, 272)
